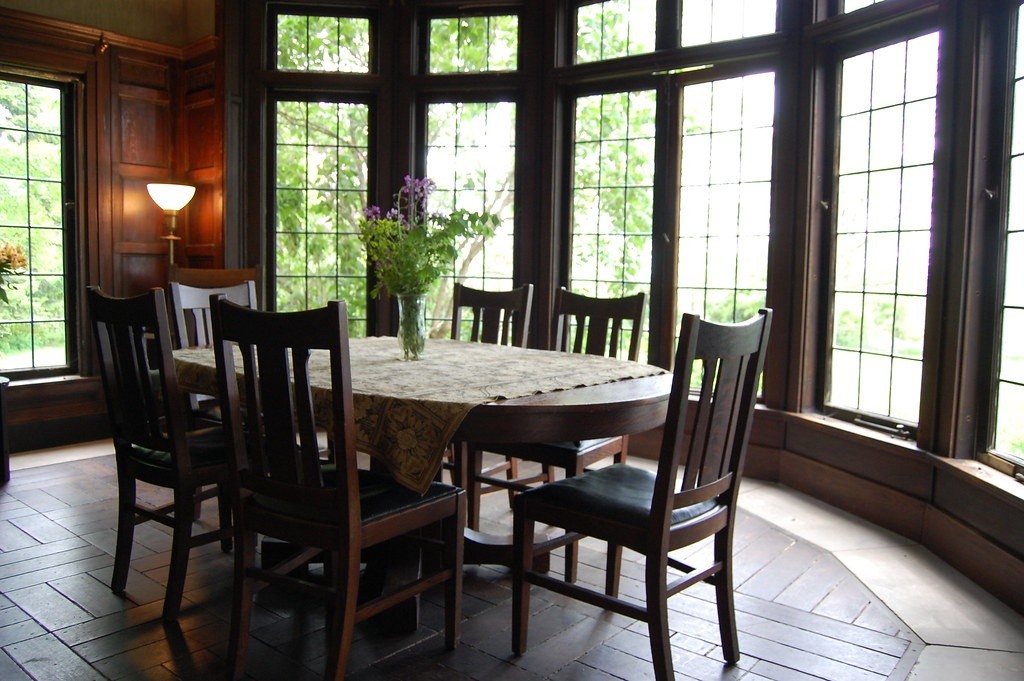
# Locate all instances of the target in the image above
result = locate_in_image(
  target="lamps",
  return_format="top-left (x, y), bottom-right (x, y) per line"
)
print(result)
top-left (146, 183), bottom-right (196, 265)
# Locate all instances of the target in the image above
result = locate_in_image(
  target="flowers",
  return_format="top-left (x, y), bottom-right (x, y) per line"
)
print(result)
top-left (356, 174), bottom-right (504, 361)
top-left (0, 238), bottom-right (28, 306)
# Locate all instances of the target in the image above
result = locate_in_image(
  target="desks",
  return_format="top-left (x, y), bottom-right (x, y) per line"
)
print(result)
top-left (171, 335), bottom-right (675, 636)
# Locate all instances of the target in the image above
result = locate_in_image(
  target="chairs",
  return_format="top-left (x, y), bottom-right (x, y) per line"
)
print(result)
top-left (509, 307), bottom-right (773, 681)
top-left (465, 285), bottom-right (648, 598)
top-left (86, 283), bottom-right (298, 625)
top-left (208, 291), bottom-right (468, 681)
top-left (430, 282), bottom-right (532, 510)
top-left (166, 278), bottom-right (266, 526)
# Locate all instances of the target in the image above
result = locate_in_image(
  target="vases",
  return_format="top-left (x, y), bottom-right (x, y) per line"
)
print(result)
top-left (395, 291), bottom-right (428, 361)
